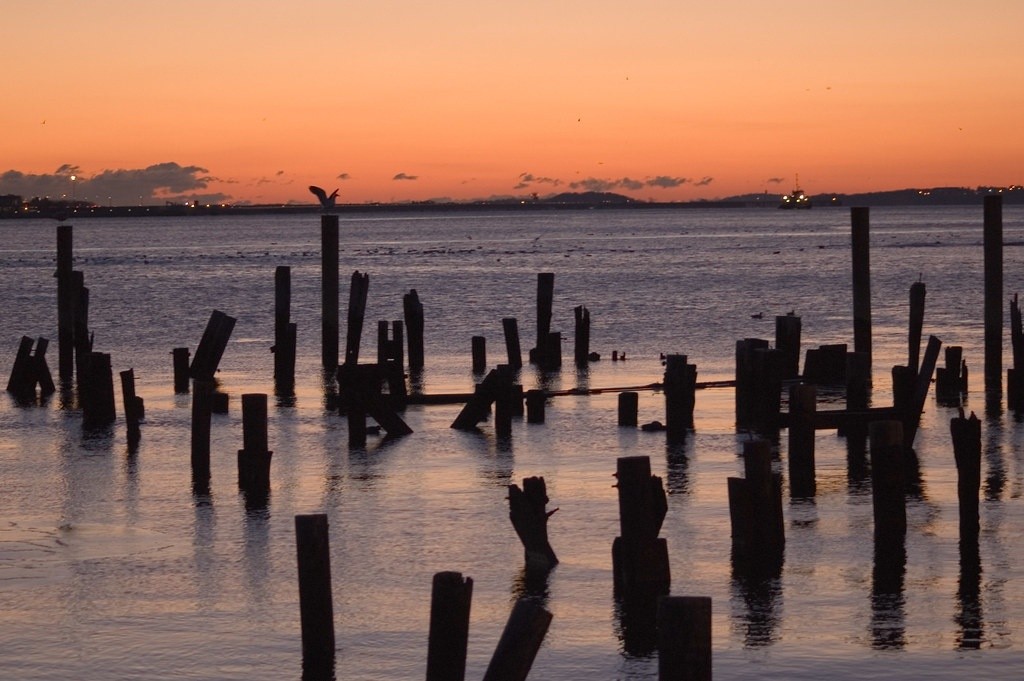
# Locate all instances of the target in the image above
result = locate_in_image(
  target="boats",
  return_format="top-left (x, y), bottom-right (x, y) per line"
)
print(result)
top-left (780, 189), bottom-right (812, 210)
